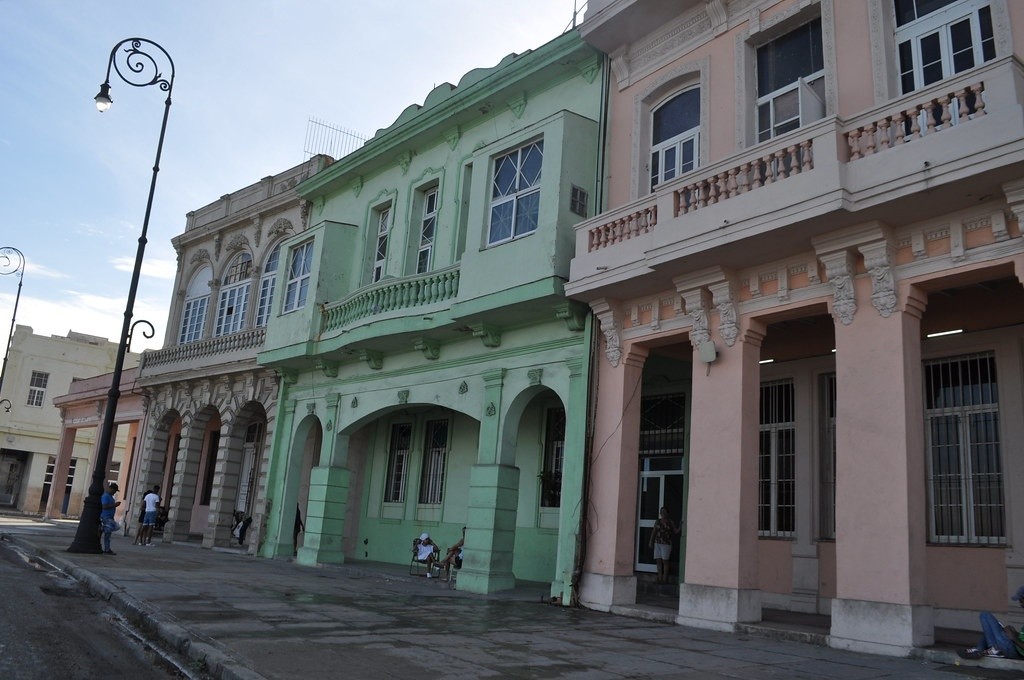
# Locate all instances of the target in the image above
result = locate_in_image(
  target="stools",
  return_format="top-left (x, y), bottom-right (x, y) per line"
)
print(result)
top-left (449, 564), bottom-right (460, 582)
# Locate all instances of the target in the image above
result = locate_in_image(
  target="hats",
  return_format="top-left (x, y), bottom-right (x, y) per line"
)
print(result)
top-left (420, 532), bottom-right (428, 540)
top-left (110, 483), bottom-right (120, 493)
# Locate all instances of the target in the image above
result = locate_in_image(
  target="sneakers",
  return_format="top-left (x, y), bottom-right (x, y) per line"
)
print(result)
top-left (982, 647), bottom-right (1005, 657)
top-left (957, 647), bottom-right (982, 658)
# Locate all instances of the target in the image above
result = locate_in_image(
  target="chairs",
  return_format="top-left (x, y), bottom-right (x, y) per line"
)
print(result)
top-left (410, 538), bottom-right (441, 579)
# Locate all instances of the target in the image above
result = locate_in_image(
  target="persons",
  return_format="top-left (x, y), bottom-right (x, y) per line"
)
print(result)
top-left (648, 506), bottom-right (683, 585)
top-left (97, 483), bottom-right (120, 556)
top-left (435, 527), bottom-right (466, 582)
top-left (131, 485), bottom-right (166, 546)
top-left (412, 533), bottom-right (439, 578)
top-left (232, 509), bottom-right (252, 547)
top-left (295, 502), bottom-right (304, 556)
top-left (971, 584), bottom-right (1024, 657)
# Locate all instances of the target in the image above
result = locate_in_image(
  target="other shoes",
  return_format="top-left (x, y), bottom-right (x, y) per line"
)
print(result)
top-left (653, 579), bottom-right (668, 585)
top-left (234, 542), bottom-right (242, 547)
top-left (106, 550), bottom-right (117, 555)
top-left (441, 578), bottom-right (448, 582)
top-left (139, 543), bottom-right (143, 546)
top-left (426, 572), bottom-right (432, 579)
top-left (434, 563), bottom-right (440, 570)
top-left (146, 543), bottom-right (155, 546)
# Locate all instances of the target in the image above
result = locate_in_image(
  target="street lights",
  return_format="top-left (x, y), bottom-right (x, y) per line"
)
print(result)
top-left (64, 35), bottom-right (177, 555)
